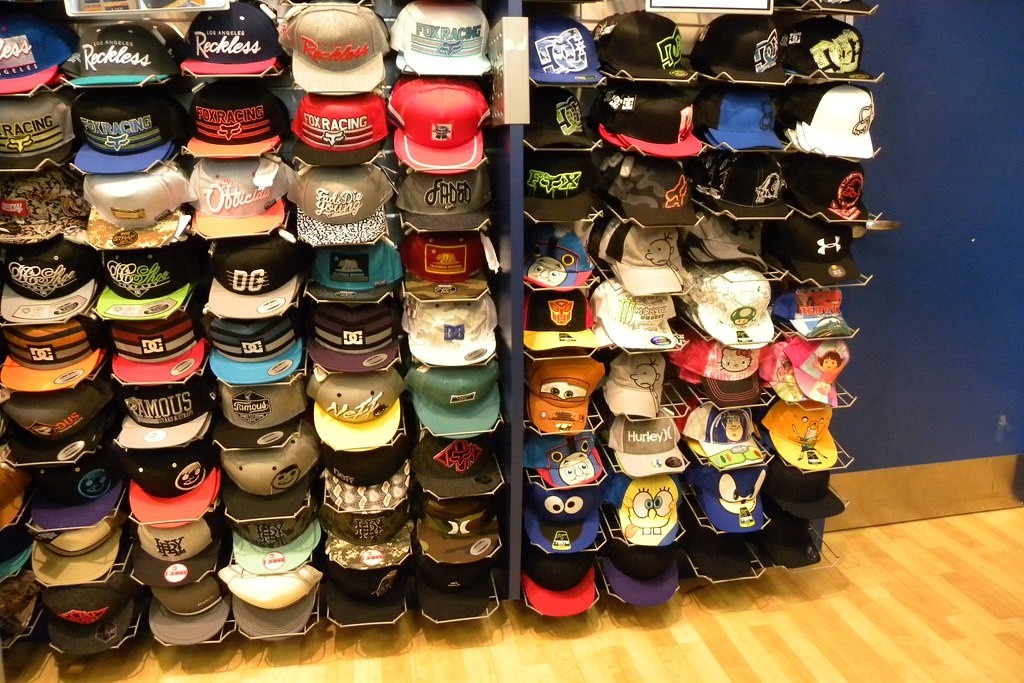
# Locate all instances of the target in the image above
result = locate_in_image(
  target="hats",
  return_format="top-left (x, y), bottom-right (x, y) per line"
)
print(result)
top-left (0, 1), bottom-right (878, 657)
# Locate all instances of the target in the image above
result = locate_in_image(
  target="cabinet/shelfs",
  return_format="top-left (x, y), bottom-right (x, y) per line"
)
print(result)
top-left (486, 1), bottom-right (904, 619)
top-left (0, 0), bottom-right (528, 653)
